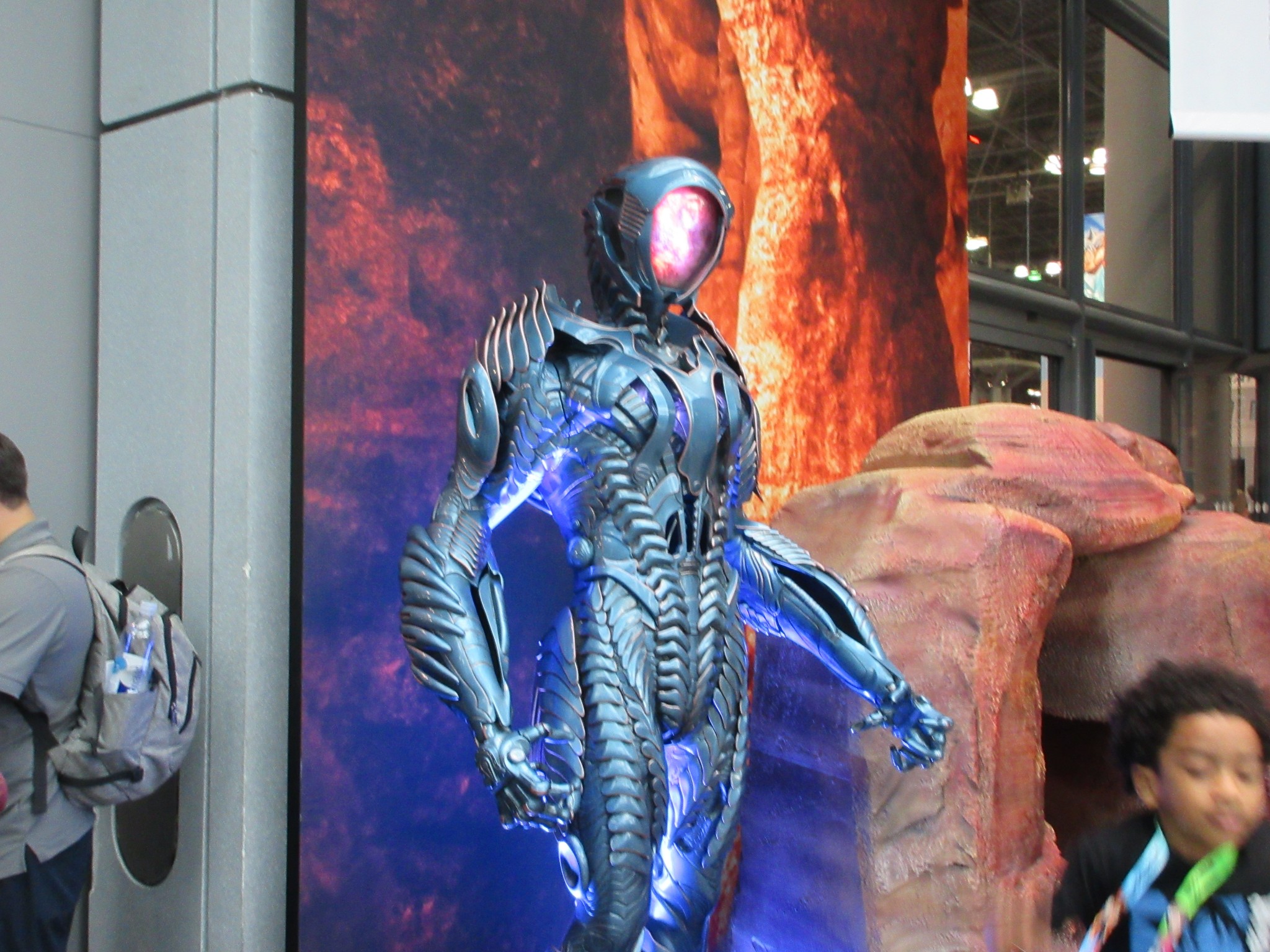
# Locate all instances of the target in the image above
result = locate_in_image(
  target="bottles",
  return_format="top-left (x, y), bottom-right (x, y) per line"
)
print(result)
top-left (107, 601), bottom-right (158, 693)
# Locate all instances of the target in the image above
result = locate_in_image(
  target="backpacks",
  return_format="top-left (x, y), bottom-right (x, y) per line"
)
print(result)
top-left (0, 526), bottom-right (203, 814)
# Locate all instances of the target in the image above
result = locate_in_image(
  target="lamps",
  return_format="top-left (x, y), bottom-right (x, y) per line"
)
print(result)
top-left (964, 74), bottom-right (1107, 281)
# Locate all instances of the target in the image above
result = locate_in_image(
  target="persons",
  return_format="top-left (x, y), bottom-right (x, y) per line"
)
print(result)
top-left (0, 433), bottom-right (109, 952)
top-left (1053, 656), bottom-right (1269, 952)
top-left (395, 151), bottom-right (956, 952)
